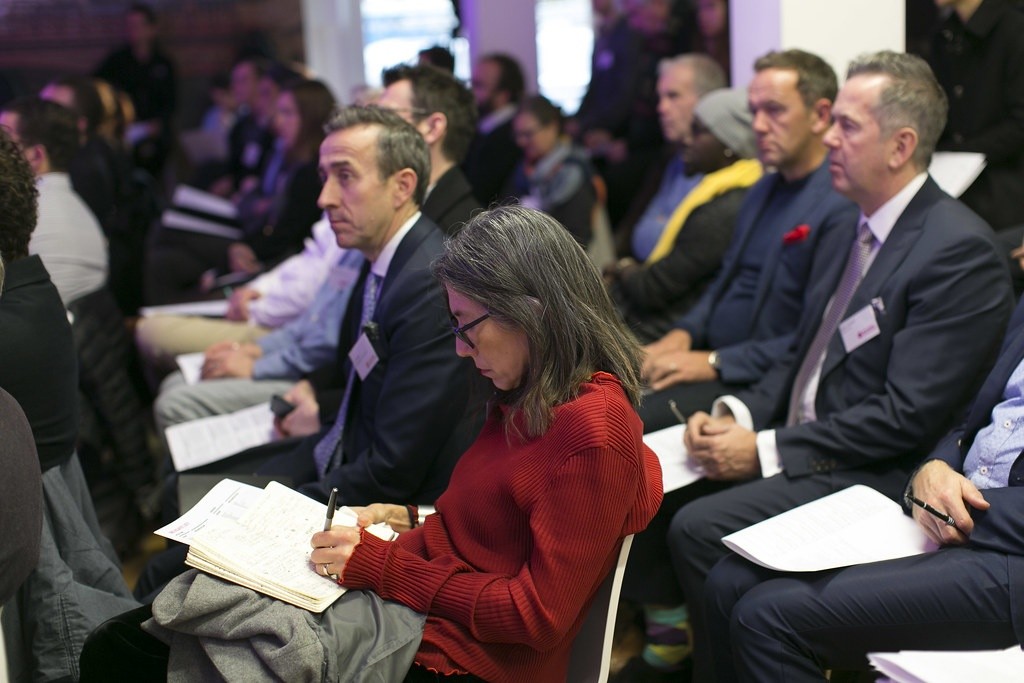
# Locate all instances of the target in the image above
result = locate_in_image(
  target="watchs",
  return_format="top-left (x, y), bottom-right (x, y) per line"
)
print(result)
top-left (709, 350), bottom-right (722, 377)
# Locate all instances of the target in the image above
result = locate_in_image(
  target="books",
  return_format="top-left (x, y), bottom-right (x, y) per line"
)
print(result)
top-left (183, 479), bottom-right (399, 615)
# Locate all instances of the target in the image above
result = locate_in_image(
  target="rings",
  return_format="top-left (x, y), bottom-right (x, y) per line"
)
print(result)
top-left (323, 563), bottom-right (329, 576)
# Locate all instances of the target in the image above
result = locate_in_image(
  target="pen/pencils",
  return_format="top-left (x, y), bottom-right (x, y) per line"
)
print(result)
top-left (903, 491), bottom-right (959, 529)
top-left (668, 399), bottom-right (687, 427)
top-left (324, 487), bottom-right (338, 531)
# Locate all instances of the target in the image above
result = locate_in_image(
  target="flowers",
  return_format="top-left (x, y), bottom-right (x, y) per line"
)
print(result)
top-left (782, 225), bottom-right (810, 244)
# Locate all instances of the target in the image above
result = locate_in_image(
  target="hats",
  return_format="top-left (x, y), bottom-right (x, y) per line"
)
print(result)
top-left (689, 85), bottom-right (759, 163)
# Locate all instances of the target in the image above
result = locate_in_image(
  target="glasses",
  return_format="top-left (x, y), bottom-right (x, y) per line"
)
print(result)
top-left (448, 312), bottom-right (494, 349)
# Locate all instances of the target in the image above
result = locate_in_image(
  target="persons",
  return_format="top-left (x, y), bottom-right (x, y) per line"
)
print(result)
top-left (78, 207), bottom-right (664, 683)
top-left (0, 0), bottom-right (1024, 683)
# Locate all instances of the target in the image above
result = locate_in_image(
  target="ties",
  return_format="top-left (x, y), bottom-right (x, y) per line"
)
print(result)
top-left (311, 270), bottom-right (379, 484)
top-left (785, 222), bottom-right (876, 424)
